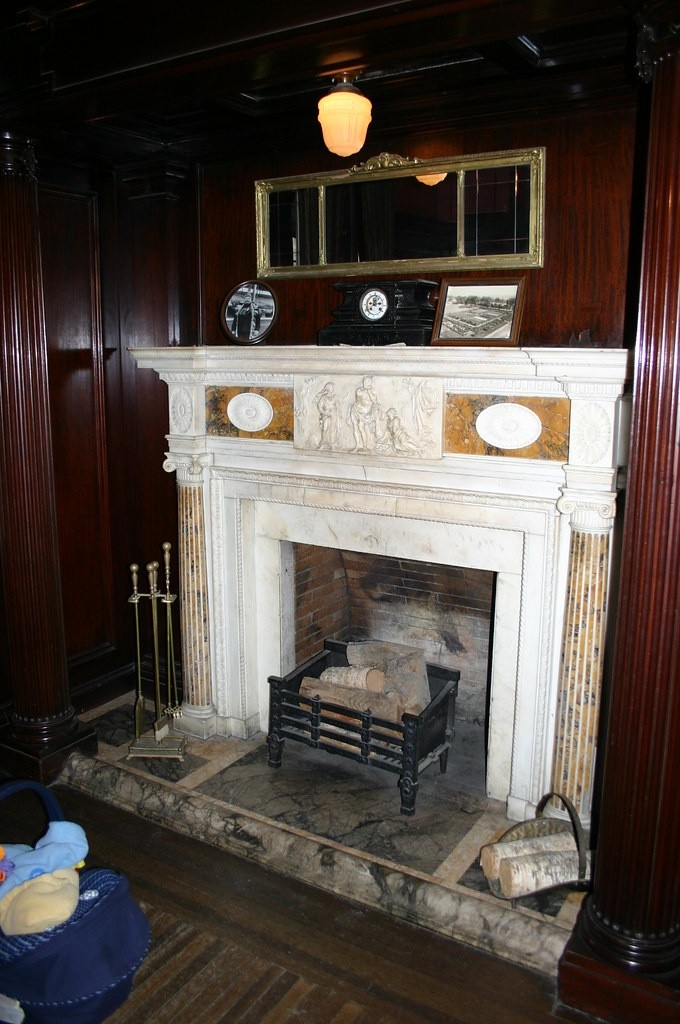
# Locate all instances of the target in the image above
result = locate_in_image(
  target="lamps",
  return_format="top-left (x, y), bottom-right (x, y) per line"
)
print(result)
top-left (316, 73), bottom-right (372, 157)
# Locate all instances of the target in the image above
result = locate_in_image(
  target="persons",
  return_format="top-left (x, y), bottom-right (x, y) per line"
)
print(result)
top-left (316, 375), bottom-right (422, 453)
top-left (231, 295), bottom-right (260, 340)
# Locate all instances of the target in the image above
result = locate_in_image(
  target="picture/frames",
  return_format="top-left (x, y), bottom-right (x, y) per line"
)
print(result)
top-left (431, 276), bottom-right (531, 348)
top-left (220, 279), bottom-right (278, 345)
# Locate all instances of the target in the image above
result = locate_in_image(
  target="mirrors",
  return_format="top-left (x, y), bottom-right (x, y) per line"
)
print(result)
top-left (255, 144), bottom-right (546, 279)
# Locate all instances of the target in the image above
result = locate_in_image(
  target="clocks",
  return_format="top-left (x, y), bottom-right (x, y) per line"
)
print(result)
top-left (317, 279), bottom-right (440, 346)
